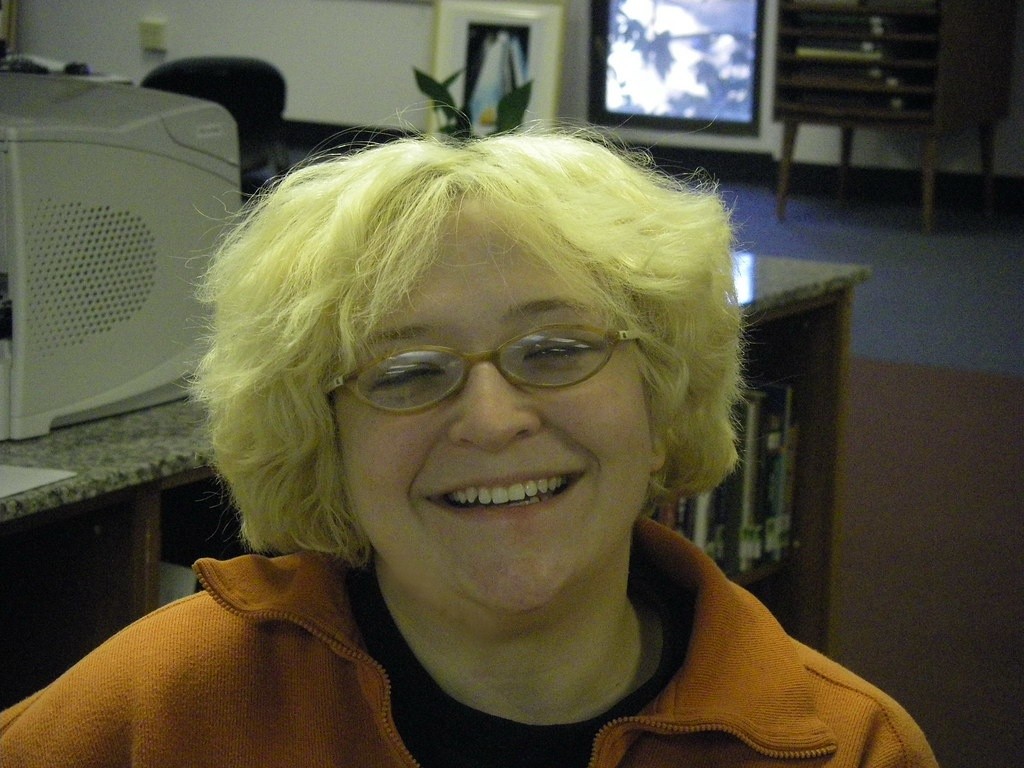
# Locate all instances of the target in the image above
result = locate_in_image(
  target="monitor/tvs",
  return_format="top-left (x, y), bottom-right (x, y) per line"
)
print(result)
top-left (0, 70), bottom-right (247, 439)
top-left (561, 0), bottom-right (780, 154)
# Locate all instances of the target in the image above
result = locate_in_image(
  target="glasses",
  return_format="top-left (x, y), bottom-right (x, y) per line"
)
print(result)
top-left (322, 323), bottom-right (642, 413)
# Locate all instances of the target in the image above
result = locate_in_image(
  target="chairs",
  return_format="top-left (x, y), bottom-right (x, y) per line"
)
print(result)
top-left (132, 53), bottom-right (291, 204)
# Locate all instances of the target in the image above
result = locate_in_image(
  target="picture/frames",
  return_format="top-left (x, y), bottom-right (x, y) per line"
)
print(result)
top-left (427, 0), bottom-right (570, 138)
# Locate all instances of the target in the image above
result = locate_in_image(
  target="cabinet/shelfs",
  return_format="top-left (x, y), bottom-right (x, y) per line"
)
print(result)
top-left (771, 0), bottom-right (1020, 235)
top-left (1, 260), bottom-right (875, 717)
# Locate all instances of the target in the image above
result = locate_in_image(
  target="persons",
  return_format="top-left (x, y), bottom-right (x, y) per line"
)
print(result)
top-left (0, 97), bottom-right (937, 768)
